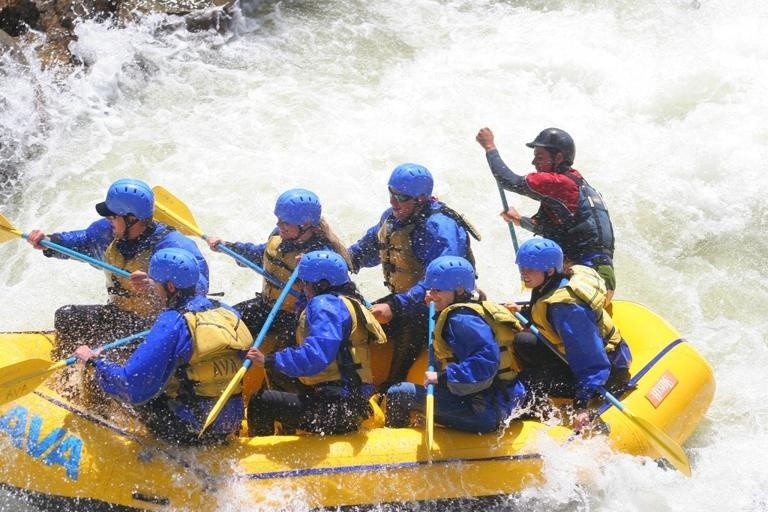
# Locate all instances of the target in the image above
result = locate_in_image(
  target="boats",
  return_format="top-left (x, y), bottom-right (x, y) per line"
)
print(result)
top-left (0, 301), bottom-right (715, 510)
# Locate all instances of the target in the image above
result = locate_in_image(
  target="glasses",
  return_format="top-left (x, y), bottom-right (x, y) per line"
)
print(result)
top-left (389, 187), bottom-right (417, 202)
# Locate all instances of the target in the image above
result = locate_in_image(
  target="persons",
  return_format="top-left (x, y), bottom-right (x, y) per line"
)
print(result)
top-left (75, 246), bottom-right (255, 447)
top-left (244, 250), bottom-right (387, 436)
top-left (476, 126), bottom-right (615, 308)
top-left (27, 177), bottom-right (210, 360)
top-left (504, 238), bottom-right (632, 430)
top-left (384, 256), bottom-right (528, 434)
top-left (205, 188), bottom-right (337, 378)
top-left (346, 162), bottom-right (476, 391)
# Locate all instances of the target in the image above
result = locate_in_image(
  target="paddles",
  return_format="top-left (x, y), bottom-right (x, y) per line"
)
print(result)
top-left (199, 265), bottom-right (298, 439)
top-left (514, 312), bottom-right (690, 478)
top-left (424, 302), bottom-right (442, 467)
top-left (0, 331), bottom-right (151, 405)
top-left (153, 186), bottom-right (303, 298)
top-left (1, 214), bottom-right (131, 280)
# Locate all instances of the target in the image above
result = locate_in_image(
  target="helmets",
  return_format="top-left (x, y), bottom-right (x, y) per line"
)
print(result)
top-left (388, 162), bottom-right (434, 197)
top-left (273, 189), bottom-right (322, 226)
top-left (105, 176), bottom-right (154, 226)
top-left (424, 254), bottom-right (476, 294)
top-left (297, 250), bottom-right (351, 289)
top-left (514, 239), bottom-right (565, 275)
top-left (148, 247), bottom-right (200, 290)
top-left (526, 127), bottom-right (576, 165)
